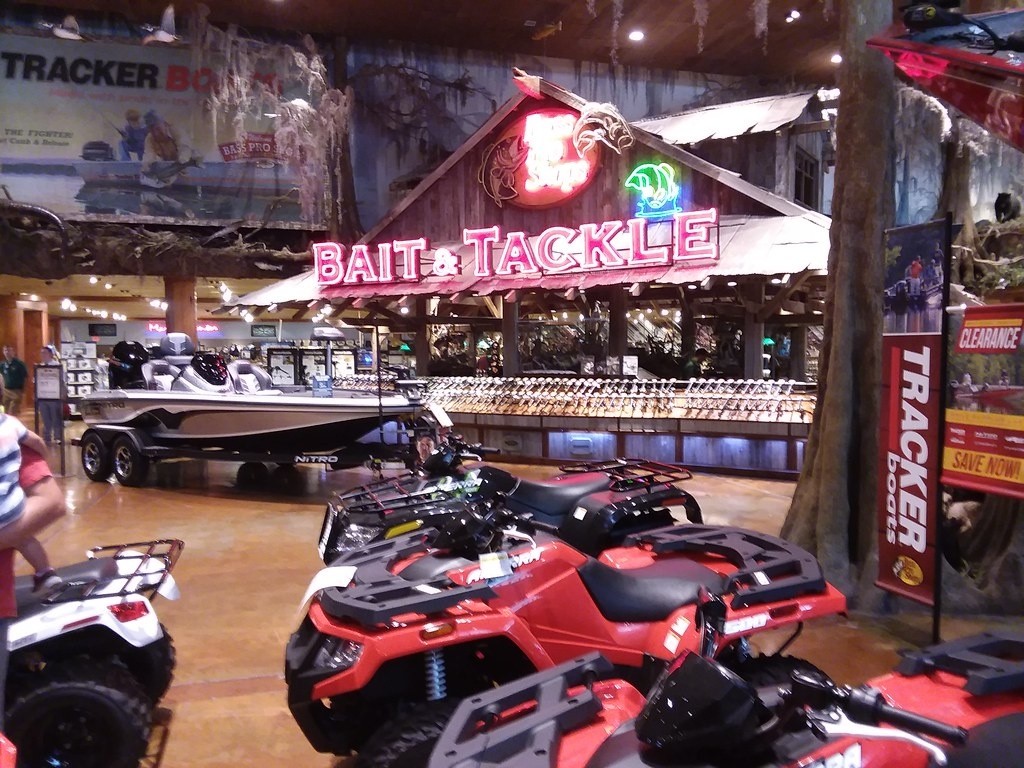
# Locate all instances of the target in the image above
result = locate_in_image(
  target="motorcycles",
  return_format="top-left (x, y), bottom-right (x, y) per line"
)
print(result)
top-left (4, 535), bottom-right (185, 768)
top-left (284, 426), bottom-right (1024, 768)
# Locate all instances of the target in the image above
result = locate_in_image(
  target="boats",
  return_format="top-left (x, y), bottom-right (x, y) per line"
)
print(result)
top-left (70, 140), bottom-right (317, 204)
top-left (881, 264), bottom-right (943, 308)
top-left (78, 350), bottom-right (416, 458)
top-left (947, 380), bottom-right (1024, 403)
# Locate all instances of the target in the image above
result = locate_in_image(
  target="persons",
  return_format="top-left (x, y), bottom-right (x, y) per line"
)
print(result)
top-left (0, 374), bottom-right (66, 728)
top-left (33, 347), bottom-right (67, 445)
top-left (681, 348), bottom-right (709, 379)
top-left (532, 339), bottom-right (548, 367)
top-left (0, 345), bottom-right (28, 417)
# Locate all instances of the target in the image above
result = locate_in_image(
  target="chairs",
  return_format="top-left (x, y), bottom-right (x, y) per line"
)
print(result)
top-left (228, 360), bottom-right (283, 396)
top-left (142, 360), bottom-right (182, 391)
